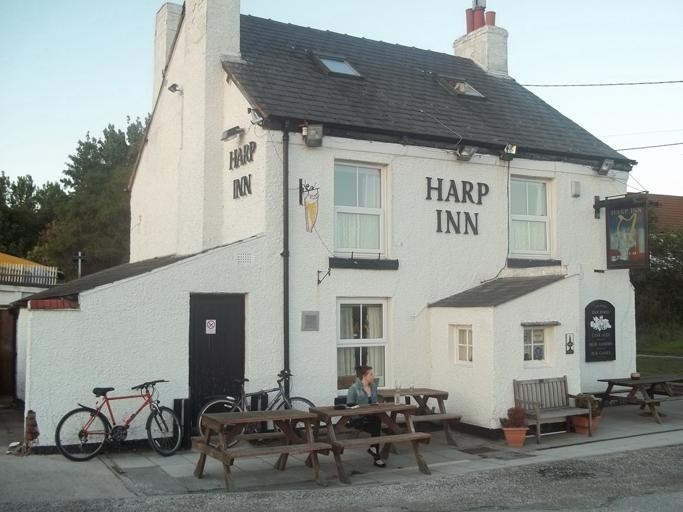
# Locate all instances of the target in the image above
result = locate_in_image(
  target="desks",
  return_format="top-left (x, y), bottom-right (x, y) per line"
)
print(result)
top-left (376, 386), bottom-right (450, 449)
top-left (309, 401), bottom-right (432, 486)
top-left (193, 409), bottom-right (331, 489)
top-left (595, 373), bottom-right (683, 425)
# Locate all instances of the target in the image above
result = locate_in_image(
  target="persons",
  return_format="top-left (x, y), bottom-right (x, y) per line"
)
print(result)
top-left (345, 364), bottom-right (385, 468)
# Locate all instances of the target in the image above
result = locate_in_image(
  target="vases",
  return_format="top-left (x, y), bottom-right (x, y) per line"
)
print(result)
top-left (502, 426), bottom-right (530, 448)
top-left (571, 414), bottom-right (601, 435)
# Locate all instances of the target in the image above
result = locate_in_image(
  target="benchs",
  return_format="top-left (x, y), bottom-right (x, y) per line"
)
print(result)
top-left (593, 388), bottom-right (683, 426)
top-left (190, 431), bottom-right (334, 489)
top-left (404, 411), bottom-right (463, 448)
top-left (318, 420), bottom-right (433, 481)
top-left (512, 375), bottom-right (594, 444)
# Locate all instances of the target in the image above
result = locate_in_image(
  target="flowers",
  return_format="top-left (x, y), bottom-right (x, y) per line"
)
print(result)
top-left (501, 405), bottom-right (529, 428)
top-left (574, 392), bottom-right (600, 416)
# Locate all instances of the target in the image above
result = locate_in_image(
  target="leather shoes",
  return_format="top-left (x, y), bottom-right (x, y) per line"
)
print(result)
top-left (368, 446), bottom-right (378, 456)
top-left (374, 459), bottom-right (385, 467)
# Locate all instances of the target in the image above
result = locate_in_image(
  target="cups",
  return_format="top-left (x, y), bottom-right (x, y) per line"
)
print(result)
top-left (409, 383), bottom-right (415, 391)
top-left (395, 383), bottom-right (401, 391)
top-left (631, 373), bottom-right (640, 380)
top-left (394, 394), bottom-right (400, 405)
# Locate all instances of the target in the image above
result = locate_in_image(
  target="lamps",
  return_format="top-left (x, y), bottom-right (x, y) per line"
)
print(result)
top-left (221, 125), bottom-right (245, 143)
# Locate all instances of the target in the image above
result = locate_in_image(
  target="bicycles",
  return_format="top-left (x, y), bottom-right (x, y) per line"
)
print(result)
top-left (197, 369), bottom-right (322, 450)
top-left (55, 379), bottom-right (184, 464)
